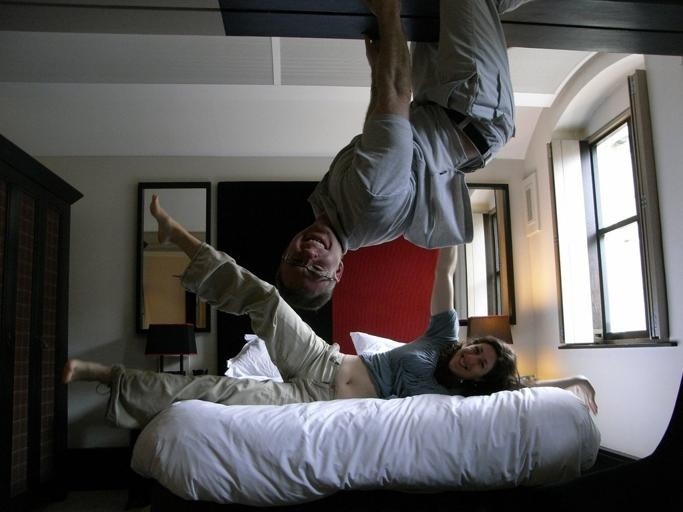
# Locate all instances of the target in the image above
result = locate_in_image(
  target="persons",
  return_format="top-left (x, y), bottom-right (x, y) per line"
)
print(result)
top-left (275, 0), bottom-right (517, 312)
top-left (57, 193), bottom-right (599, 432)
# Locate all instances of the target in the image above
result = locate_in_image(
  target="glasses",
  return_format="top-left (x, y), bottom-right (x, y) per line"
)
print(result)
top-left (281, 254), bottom-right (334, 283)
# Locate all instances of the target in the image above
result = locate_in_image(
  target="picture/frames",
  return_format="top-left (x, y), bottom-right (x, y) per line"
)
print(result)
top-left (522, 172), bottom-right (540, 238)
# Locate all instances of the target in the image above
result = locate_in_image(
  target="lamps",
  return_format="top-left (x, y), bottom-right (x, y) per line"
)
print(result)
top-left (467, 316), bottom-right (514, 345)
top-left (145, 323), bottom-right (197, 376)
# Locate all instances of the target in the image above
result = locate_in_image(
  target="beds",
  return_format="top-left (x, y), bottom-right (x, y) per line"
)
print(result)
top-left (130, 386), bottom-right (601, 512)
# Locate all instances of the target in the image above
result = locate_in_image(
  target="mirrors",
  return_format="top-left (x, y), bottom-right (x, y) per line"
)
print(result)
top-left (455, 183), bottom-right (516, 325)
top-left (136, 181), bottom-right (211, 338)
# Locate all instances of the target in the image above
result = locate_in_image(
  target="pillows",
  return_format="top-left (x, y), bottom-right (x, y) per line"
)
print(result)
top-left (350, 331), bottom-right (403, 355)
top-left (224, 334), bottom-right (281, 379)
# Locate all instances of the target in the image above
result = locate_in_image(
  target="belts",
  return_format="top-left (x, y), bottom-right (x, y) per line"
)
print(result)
top-left (450, 109), bottom-right (493, 167)
